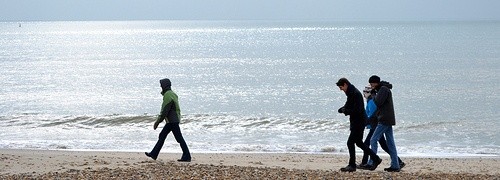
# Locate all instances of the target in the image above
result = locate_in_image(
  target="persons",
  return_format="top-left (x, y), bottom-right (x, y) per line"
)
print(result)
top-left (356, 85), bottom-right (406, 171)
top-left (336, 78), bottom-right (382, 172)
top-left (145, 79), bottom-right (191, 162)
top-left (364, 75), bottom-right (401, 172)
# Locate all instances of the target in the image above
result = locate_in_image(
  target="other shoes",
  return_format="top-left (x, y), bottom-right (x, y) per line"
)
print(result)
top-left (384, 166), bottom-right (400, 172)
top-left (399, 162), bottom-right (405, 169)
top-left (145, 152), bottom-right (156, 160)
top-left (356, 164), bottom-right (365, 169)
top-left (341, 166), bottom-right (356, 172)
top-left (364, 164), bottom-right (372, 170)
top-left (371, 158), bottom-right (382, 171)
top-left (177, 159), bottom-right (185, 162)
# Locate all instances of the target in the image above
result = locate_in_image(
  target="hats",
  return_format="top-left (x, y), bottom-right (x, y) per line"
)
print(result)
top-left (369, 75), bottom-right (380, 83)
top-left (363, 84), bottom-right (372, 98)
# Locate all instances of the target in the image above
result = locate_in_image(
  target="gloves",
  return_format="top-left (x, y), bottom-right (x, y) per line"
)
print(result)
top-left (338, 107), bottom-right (344, 113)
top-left (370, 89), bottom-right (377, 95)
top-left (154, 122), bottom-right (159, 130)
